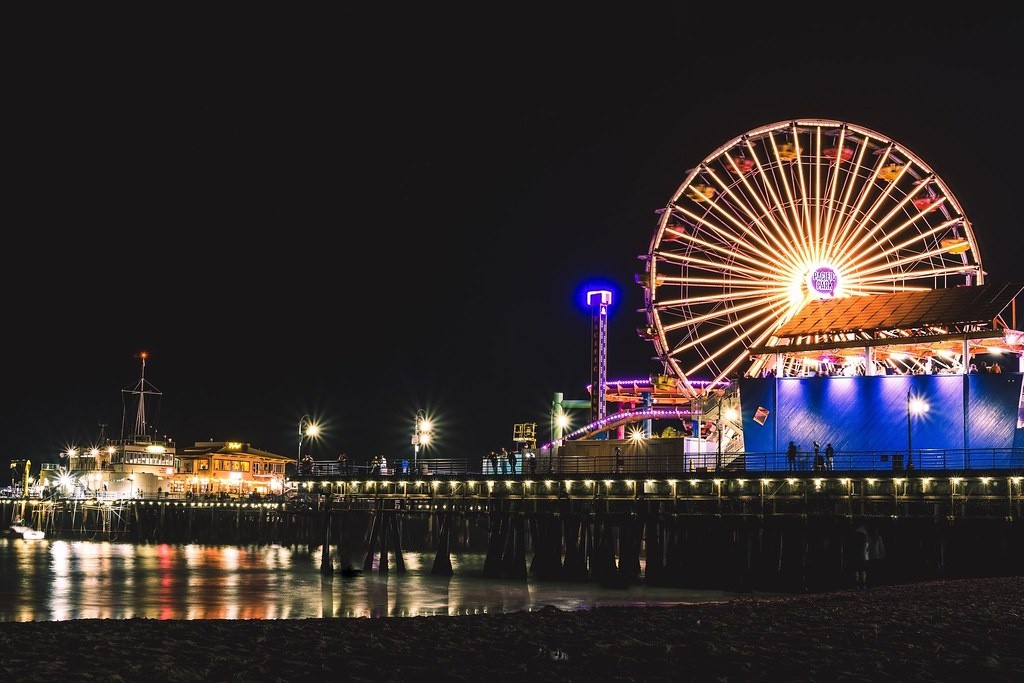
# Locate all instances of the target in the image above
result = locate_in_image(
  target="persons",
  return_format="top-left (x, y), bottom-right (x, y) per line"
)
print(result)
top-left (42, 483), bottom-right (107, 497)
top-left (102, 459), bottom-right (107, 469)
top-left (787, 440), bottom-right (838, 473)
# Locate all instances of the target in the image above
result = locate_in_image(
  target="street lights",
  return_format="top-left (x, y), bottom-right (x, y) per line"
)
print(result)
top-left (906, 384), bottom-right (928, 470)
top-left (716, 394), bottom-right (736, 471)
top-left (962, 331), bottom-right (1002, 470)
top-left (297, 415), bottom-right (318, 475)
top-left (548, 401), bottom-right (569, 472)
top-left (412, 409), bottom-right (433, 475)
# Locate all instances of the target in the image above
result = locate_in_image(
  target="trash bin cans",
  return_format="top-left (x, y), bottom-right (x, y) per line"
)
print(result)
top-left (892, 454), bottom-right (904, 469)
